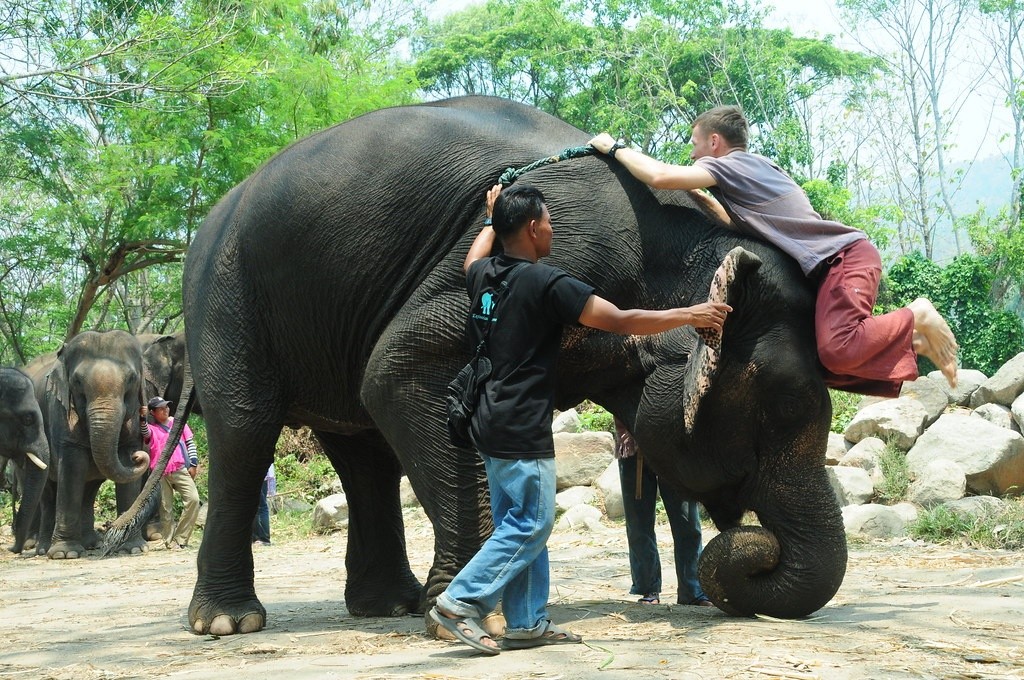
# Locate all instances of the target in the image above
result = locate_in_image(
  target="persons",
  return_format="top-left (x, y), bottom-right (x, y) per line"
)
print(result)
top-left (252, 464), bottom-right (276, 545)
top-left (139, 397), bottom-right (199, 549)
top-left (614, 417), bottom-right (715, 606)
top-left (587, 104), bottom-right (958, 398)
top-left (429, 185), bottom-right (733, 653)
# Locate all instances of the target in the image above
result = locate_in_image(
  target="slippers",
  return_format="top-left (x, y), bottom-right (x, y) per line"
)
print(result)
top-left (639, 597), bottom-right (659, 606)
top-left (502, 620), bottom-right (582, 648)
top-left (428, 605), bottom-right (502, 655)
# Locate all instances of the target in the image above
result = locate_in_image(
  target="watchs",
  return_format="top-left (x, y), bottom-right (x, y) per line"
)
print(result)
top-left (484, 219), bottom-right (492, 226)
top-left (609, 142), bottom-right (627, 158)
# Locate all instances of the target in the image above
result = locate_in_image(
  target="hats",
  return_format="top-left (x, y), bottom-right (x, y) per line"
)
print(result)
top-left (148, 396), bottom-right (173, 410)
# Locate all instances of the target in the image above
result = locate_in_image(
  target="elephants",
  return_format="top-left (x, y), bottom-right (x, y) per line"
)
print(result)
top-left (0, 330), bottom-right (203, 562)
top-left (103, 94), bottom-right (849, 635)
